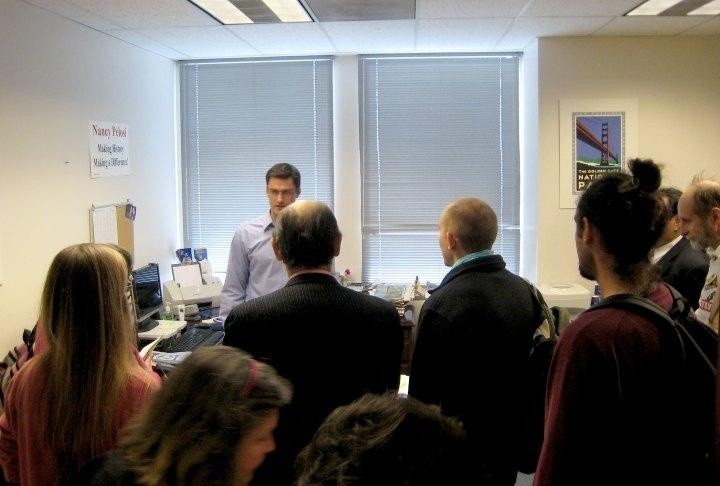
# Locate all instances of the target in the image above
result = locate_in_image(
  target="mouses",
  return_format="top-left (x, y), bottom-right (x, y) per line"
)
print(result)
top-left (212, 317), bottom-right (225, 330)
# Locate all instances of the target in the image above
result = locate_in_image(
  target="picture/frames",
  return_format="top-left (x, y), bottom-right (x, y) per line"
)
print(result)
top-left (558, 101), bottom-right (638, 209)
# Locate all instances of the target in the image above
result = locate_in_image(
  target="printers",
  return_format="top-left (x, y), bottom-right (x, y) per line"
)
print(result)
top-left (163, 260), bottom-right (225, 326)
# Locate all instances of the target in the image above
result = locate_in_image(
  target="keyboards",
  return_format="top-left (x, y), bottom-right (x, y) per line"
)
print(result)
top-left (160, 328), bottom-right (225, 353)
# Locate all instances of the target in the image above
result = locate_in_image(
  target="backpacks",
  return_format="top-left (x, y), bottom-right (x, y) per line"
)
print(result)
top-left (585, 283), bottom-right (718, 485)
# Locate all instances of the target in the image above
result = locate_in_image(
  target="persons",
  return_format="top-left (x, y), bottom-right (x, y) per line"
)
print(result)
top-left (294, 390), bottom-right (470, 485)
top-left (409, 198), bottom-right (553, 486)
top-left (94, 343), bottom-right (295, 485)
top-left (224, 197), bottom-right (403, 486)
top-left (32, 242), bottom-right (134, 358)
top-left (677, 170), bottom-right (720, 333)
top-left (0, 242), bottom-right (164, 485)
top-left (534, 159), bottom-right (720, 485)
top-left (217, 164), bottom-right (336, 326)
top-left (644, 186), bottom-right (708, 311)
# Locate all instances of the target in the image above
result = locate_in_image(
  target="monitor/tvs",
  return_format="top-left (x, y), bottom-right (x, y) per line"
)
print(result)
top-left (130, 263), bottom-right (164, 332)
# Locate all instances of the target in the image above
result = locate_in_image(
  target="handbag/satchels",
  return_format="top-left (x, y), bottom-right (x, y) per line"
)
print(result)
top-left (0, 330), bottom-right (35, 415)
top-left (519, 278), bottom-right (569, 475)
